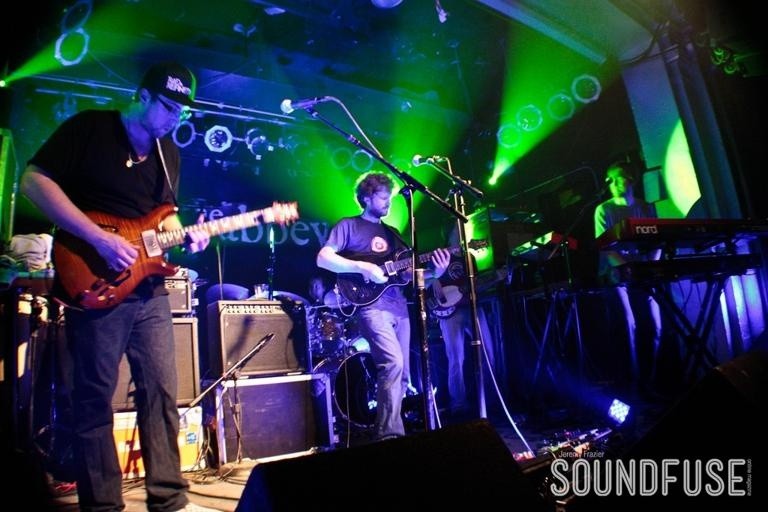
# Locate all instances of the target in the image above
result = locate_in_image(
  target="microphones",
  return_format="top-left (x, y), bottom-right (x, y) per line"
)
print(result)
top-left (280, 97), bottom-right (321, 115)
top-left (413, 155), bottom-right (443, 167)
top-left (596, 177), bottom-right (613, 199)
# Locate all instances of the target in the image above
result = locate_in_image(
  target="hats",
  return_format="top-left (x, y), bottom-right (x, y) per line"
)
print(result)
top-left (139, 62), bottom-right (202, 109)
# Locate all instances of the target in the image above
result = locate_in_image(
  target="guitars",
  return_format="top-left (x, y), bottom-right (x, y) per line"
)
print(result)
top-left (53, 201), bottom-right (302, 310)
top-left (341, 238), bottom-right (490, 307)
top-left (424, 262), bottom-right (512, 319)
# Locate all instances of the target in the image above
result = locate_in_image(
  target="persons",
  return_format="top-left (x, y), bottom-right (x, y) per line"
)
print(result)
top-left (315, 169), bottom-right (452, 444)
top-left (431, 225), bottom-right (496, 440)
top-left (18, 59), bottom-right (228, 512)
top-left (307, 273), bottom-right (327, 304)
top-left (593, 160), bottom-right (676, 417)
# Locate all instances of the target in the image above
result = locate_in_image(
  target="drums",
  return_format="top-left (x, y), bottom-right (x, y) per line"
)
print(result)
top-left (329, 352), bottom-right (379, 428)
top-left (308, 311), bottom-right (346, 357)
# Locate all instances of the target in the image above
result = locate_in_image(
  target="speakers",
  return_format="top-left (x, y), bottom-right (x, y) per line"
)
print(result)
top-left (207, 300), bottom-right (312, 380)
top-left (565, 348), bottom-right (767, 511)
top-left (236, 417), bottom-right (555, 512)
top-left (111, 317), bottom-right (201, 412)
top-left (165, 276), bottom-right (192, 314)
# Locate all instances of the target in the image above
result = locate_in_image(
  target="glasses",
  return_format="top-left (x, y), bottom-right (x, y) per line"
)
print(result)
top-left (155, 94), bottom-right (194, 121)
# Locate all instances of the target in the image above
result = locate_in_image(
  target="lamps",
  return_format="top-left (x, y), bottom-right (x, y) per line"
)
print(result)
top-left (171, 107), bottom-right (314, 164)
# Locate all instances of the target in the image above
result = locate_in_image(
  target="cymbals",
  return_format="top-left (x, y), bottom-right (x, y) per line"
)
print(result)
top-left (324, 289), bottom-right (352, 308)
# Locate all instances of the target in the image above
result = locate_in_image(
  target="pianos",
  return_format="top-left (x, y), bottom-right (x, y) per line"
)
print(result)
top-left (594, 216), bottom-right (747, 249)
top-left (597, 254), bottom-right (759, 288)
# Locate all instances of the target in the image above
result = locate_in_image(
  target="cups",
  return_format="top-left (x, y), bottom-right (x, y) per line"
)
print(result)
top-left (254, 283), bottom-right (269, 300)
